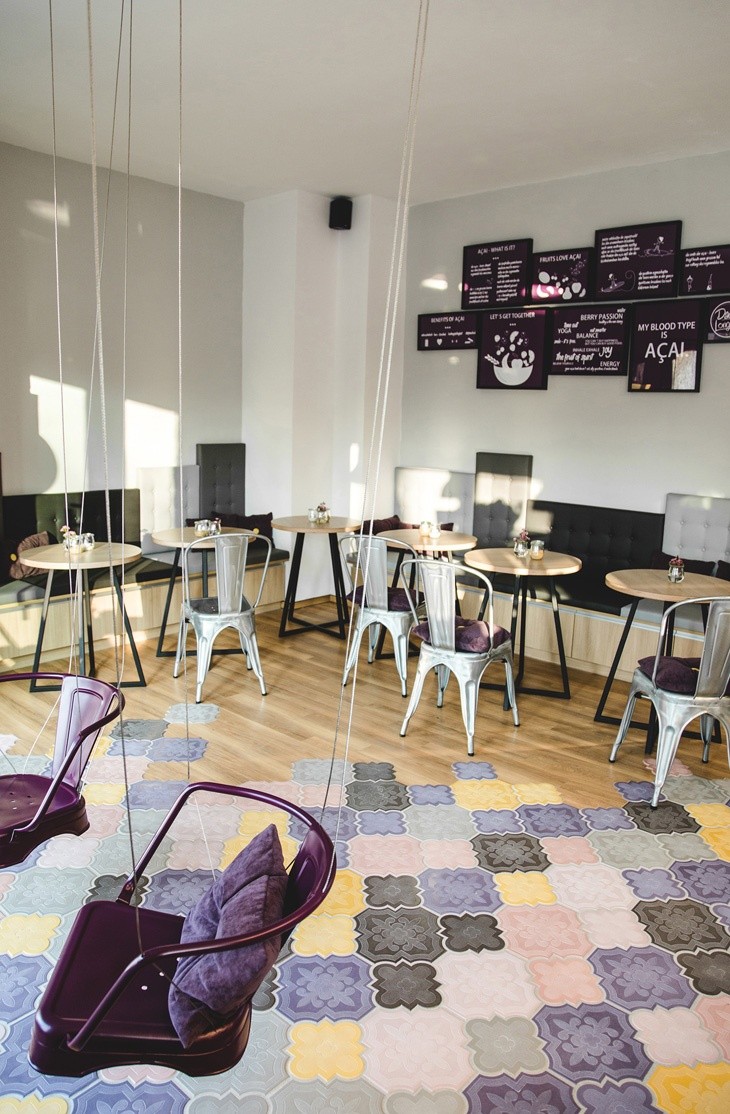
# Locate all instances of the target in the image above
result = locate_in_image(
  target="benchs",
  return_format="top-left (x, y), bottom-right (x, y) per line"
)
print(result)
top-left (0, 491), bottom-right (289, 673)
top-left (352, 466), bottom-right (730, 687)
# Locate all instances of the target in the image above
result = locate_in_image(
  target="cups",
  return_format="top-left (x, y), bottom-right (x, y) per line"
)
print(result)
top-left (308, 509), bottom-right (318, 522)
top-left (194, 520), bottom-right (221, 537)
top-left (64, 533), bottom-right (95, 553)
top-left (420, 522), bottom-right (441, 538)
top-left (530, 540), bottom-right (544, 559)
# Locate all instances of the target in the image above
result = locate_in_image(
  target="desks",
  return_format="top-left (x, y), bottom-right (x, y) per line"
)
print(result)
top-left (269, 513), bottom-right (364, 640)
top-left (594, 566), bottom-right (730, 755)
top-left (460, 546), bottom-right (583, 710)
top-left (148, 525), bottom-right (259, 656)
top-left (19, 540), bottom-right (148, 693)
top-left (375, 526), bottom-right (477, 662)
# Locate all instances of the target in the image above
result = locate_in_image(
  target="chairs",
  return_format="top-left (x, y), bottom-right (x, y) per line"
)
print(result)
top-left (394, 557), bottom-right (521, 756)
top-left (170, 532), bottom-right (273, 706)
top-left (333, 533), bottom-right (438, 699)
top-left (609, 594), bottom-right (730, 808)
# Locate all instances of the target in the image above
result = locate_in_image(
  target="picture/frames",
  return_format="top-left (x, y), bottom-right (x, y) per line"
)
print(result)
top-left (415, 220), bottom-right (730, 396)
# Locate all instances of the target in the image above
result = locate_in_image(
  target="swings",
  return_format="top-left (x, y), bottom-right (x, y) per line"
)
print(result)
top-left (30, 1), bottom-right (440, 1077)
top-left (2, 0), bottom-right (135, 874)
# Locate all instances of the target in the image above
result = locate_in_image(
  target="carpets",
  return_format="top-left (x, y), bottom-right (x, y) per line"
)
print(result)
top-left (0, 703), bottom-right (729, 1114)
